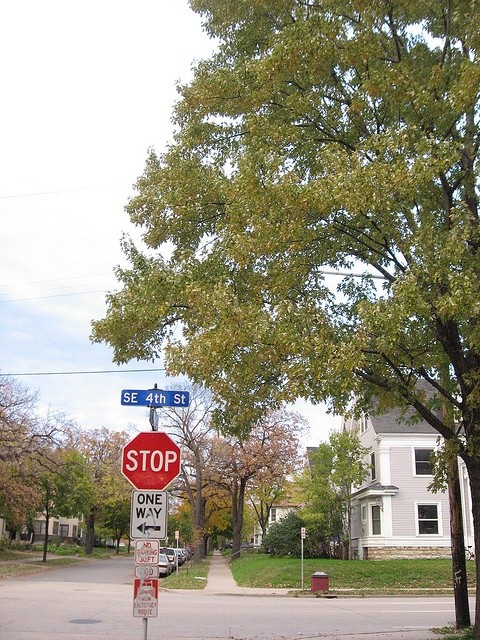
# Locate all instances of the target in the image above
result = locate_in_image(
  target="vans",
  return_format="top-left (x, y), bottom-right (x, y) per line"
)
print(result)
top-left (160, 547), bottom-right (176, 572)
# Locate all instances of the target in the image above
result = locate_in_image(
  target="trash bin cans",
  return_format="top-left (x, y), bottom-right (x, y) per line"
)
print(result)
top-left (308, 570), bottom-right (331, 592)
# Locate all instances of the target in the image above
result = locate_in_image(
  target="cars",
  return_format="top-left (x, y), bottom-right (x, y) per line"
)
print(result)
top-left (175, 548), bottom-right (186, 565)
top-left (185, 549), bottom-right (192, 560)
top-left (158, 554), bottom-right (173, 577)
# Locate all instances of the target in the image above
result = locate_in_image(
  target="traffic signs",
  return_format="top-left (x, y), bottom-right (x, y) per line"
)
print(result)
top-left (121, 432), bottom-right (181, 491)
